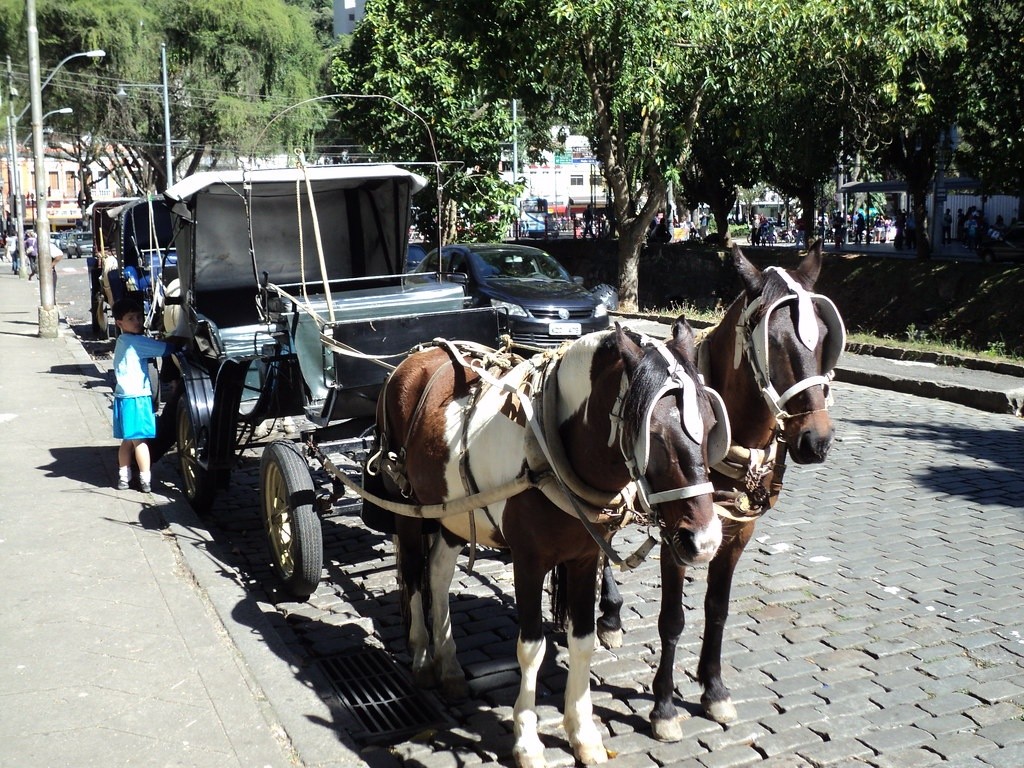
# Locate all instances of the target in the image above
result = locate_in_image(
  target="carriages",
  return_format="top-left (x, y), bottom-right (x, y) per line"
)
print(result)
top-left (84, 165), bottom-right (847, 768)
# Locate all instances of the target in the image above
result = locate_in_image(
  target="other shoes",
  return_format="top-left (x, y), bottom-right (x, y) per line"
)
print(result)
top-left (118, 478), bottom-right (139, 489)
top-left (142, 482), bottom-right (151, 492)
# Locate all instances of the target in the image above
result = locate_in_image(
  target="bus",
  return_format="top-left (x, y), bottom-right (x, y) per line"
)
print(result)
top-left (520, 198), bottom-right (548, 238)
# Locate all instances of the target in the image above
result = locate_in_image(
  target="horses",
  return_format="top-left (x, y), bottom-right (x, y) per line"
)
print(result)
top-left (595, 236), bottom-right (849, 745)
top-left (375, 313), bottom-right (725, 768)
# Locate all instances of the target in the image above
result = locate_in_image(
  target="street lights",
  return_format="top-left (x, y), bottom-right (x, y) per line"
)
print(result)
top-left (116, 43), bottom-right (172, 191)
top-left (9, 50), bottom-right (106, 280)
top-left (9, 108), bottom-right (74, 235)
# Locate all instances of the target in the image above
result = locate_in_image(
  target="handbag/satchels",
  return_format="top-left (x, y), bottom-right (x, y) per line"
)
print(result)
top-left (28, 261), bottom-right (33, 275)
top-left (25, 245), bottom-right (34, 255)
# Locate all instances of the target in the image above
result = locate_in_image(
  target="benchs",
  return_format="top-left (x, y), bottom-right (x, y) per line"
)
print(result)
top-left (186, 272), bottom-right (378, 367)
top-left (260, 269), bottom-right (466, 346)
top-left (139, 250), bottom-right (179, 289)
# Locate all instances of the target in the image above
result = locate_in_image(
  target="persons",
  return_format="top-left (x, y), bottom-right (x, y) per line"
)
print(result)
top-left (1, 230), bottom-right (19, 274)
top-left (732, 212), bottom-right (805, 246)
top-left (50, 243), bottom-right (63, 305)
top-left (649, 212), bottom-right (711, 239)
top-left (942, 209), bottom-right (952, 247)
top-left (958, 206), bottom-right (1018, 249)
top-left (111, 297), bottom-right (184, 492)
top-left (548, 214), bottom-right (607, 239)
top-left (817, 211), bottom-right (914, 248)
top-left (24, 232), bottom-right (38, 280)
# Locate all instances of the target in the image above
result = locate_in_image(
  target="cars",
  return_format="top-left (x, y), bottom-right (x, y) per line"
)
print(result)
top-left (50, 232), bottom-right (74, 252)
top-left (764, 217), bottom-right (778, 225)
top-left (66, 231), bottom-right (93, 259)
top-left (407, 243), bottom-right (427, 272)
top-left (404, 242), bottom-right (609, 361)
